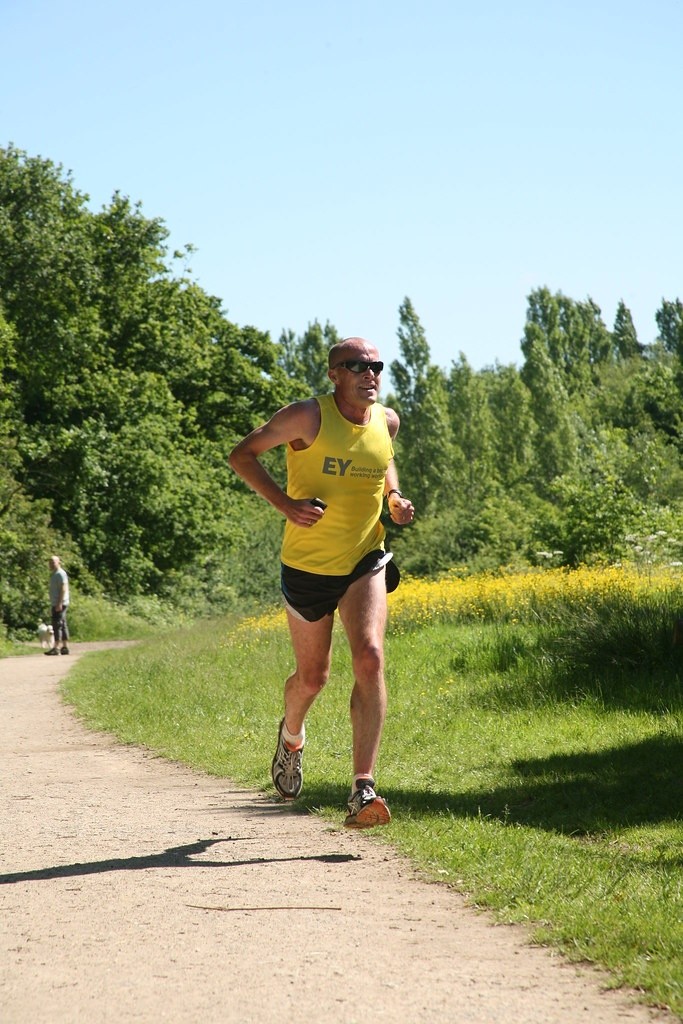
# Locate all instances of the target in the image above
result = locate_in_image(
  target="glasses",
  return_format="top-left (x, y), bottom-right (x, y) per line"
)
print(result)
top-left (332, 360), bottom-right (383, 373)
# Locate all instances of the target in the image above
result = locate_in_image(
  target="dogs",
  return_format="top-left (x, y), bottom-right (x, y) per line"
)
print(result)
top-left (37, 623), bottom-right (54, 649)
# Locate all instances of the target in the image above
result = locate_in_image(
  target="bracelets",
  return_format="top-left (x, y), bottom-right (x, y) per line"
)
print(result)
top-left (386, 489), bottom-right (403, 499)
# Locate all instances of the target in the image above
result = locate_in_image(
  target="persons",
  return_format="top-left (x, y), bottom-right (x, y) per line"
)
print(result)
top-left (228, 338), bottom-right (415, 829)
top-left (44, 555), bottom-right (70, 655)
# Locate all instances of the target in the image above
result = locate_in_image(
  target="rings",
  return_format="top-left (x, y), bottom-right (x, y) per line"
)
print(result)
top-left (306, 519), bottom-right (311, 525)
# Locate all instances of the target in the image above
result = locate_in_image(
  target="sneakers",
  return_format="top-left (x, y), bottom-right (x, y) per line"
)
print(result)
top-left (271, 717), bottom-right (305, 801)
top-left (44, 648), bottom-right (59, 656)
top-left (61, 647), bottom-right (68, 655)
top-left (342, 778), bottom-right (391, 829)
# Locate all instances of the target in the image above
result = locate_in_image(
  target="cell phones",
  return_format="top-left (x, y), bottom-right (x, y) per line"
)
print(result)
top-left (310, 497), bottom-right (328, 511)
